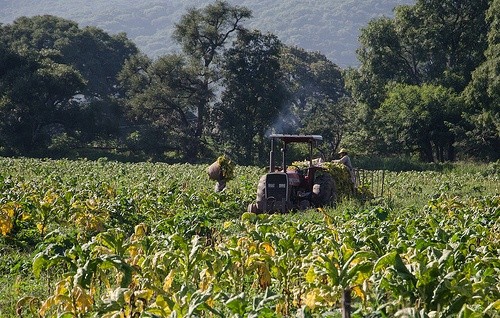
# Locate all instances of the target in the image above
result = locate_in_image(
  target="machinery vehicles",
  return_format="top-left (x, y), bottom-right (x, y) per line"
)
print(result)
top-left (247, 132), bottom-right (338, 219)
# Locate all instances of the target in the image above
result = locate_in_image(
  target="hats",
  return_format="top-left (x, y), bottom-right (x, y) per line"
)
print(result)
top-left (338, 148), bottom-right (347, 153)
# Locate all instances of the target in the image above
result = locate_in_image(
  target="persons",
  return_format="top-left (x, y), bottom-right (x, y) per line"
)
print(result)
top-left (215, 179), bottom-right (227, 194)
top-left (332, 148), bottom-right (356, 188)
